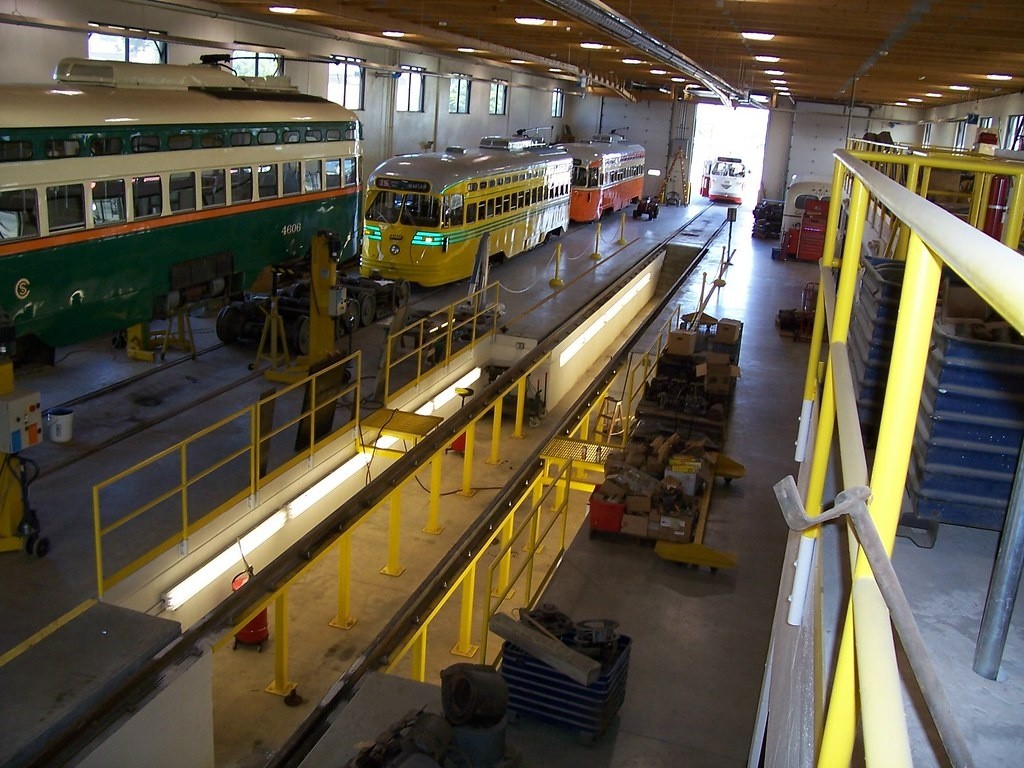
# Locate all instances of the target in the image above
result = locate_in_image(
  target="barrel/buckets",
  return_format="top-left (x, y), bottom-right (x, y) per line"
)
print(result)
top-left (45, 407), bottom-right (75, 443)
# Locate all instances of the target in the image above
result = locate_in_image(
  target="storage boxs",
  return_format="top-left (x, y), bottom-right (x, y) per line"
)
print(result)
top-left (589, 318), bottom-right (741, 543)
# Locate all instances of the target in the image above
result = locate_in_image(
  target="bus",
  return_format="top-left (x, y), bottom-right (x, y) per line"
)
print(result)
top-left (700, 156), bottom-right (749, 205)
top-left (0, 53), bottom-right (364, 360)
top-left (551, 127), bottom-right (647, 225)
top-left (358, 126), bottom-right (574, 288)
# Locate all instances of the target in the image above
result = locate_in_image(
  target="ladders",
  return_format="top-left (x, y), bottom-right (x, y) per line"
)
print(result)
top-left (654, 145), bottom-right (688, 207)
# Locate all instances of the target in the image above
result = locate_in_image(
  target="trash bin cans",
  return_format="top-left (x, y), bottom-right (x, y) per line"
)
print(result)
top-left (45, 406), bottom-right (75, 443)
